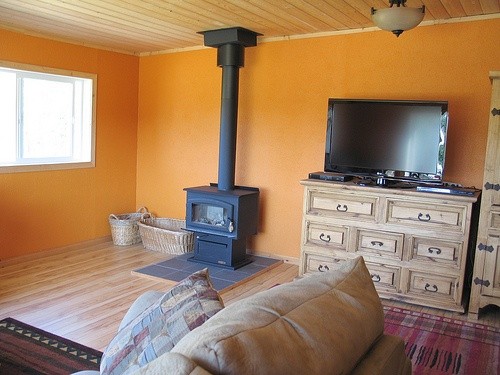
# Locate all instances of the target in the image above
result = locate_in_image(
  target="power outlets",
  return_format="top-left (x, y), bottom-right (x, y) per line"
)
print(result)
top-left (442, 181), bottom-right (462, 187)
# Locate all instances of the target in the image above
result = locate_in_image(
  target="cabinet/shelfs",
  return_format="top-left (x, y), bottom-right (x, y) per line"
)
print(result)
top-left (467, 69), bottom-right (500, 321)
top-left (292, 177), bottom-right (482, 314)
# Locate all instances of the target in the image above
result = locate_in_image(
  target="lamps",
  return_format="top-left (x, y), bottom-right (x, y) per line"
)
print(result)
top-left (371, 0), bottom-right (426, 38)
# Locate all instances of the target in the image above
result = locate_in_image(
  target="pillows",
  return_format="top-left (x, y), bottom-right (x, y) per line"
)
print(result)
top-left (99, 268), bottom-right (225, 375)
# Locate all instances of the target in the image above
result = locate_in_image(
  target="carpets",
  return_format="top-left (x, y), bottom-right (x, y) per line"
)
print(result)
top-left (267, 283), bottom-right (500, 375)
top-left (0, 317), bottom-right (104, 375)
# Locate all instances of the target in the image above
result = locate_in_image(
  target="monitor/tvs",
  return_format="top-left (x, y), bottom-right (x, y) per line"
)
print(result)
top-left (324, 99), bottom-right (449, 188)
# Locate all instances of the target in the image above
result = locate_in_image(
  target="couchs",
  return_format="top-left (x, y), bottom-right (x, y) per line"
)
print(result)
top-left (69, 254), bottom-right (413, 375)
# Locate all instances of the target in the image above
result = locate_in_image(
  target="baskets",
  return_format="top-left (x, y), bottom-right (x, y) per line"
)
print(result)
top-left (108, 207), bottom-right (155, 246)
top-left (137, 213), bottom-right (194, 255)
